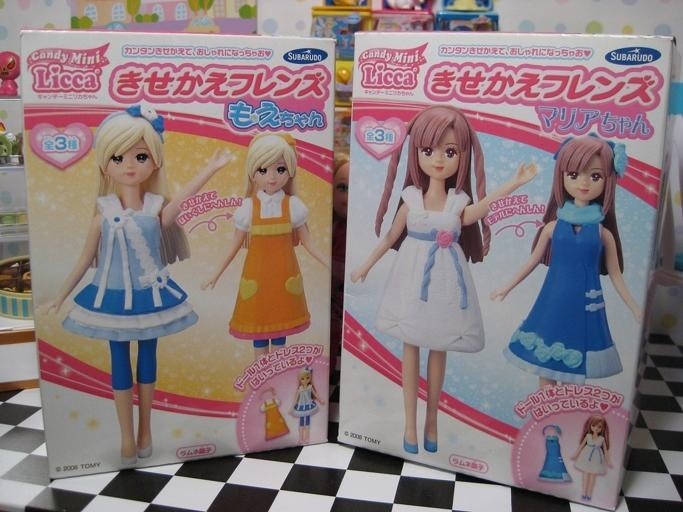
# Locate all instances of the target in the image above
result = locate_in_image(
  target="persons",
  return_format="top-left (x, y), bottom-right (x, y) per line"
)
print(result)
top-left (40, 105), bottom-right (233, 464)
top-left (289, 368), bottom-right (325, 446)
top-left (568, 412), bottom-right (614, 503)
top-left (201, 128), bottom-right (331, 360)
top-left (493, 137), bottom-right (642, 399)
top-left (350, 108), bottom-right (538, 456)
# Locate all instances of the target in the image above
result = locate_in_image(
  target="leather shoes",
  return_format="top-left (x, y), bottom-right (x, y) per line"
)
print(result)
top-left (118, 435), bottom-right (153, 468)
top-left (403, 426), bottom-right (441, 455)
